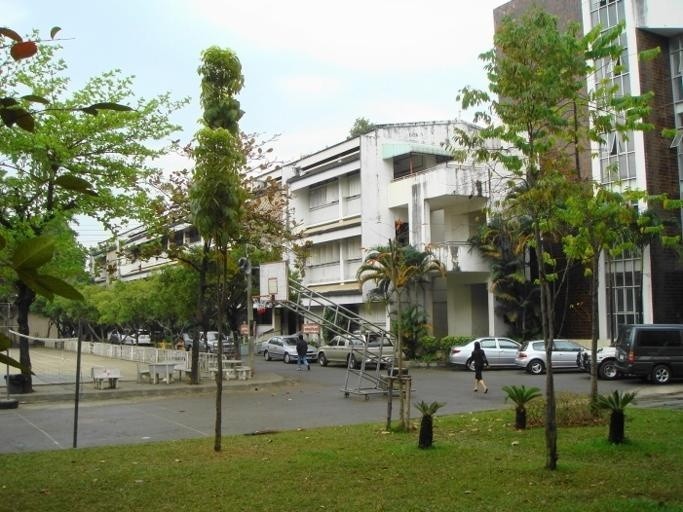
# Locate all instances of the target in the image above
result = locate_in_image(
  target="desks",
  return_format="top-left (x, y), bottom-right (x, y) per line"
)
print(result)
top-left (148, 361), bottom-right (178, 384)
top-left (208, 359), bottom-right (243, 379)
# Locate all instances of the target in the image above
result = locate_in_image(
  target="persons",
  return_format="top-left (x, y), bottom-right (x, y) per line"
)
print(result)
top-left (295, 335), bottom-right (310, 370)
top-left (468, 341), bottom-right (489, 394)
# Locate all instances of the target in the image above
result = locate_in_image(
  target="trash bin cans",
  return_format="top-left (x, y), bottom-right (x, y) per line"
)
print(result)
top-left (4, 374), bottom-right (25, 394)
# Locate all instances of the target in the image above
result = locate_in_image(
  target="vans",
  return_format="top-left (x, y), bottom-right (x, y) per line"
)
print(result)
top-left (616, 324), bottom-right (683, 385)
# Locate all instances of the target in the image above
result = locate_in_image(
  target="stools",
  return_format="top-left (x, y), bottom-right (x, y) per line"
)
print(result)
top-left (209, 364), bottom-right (252, 381)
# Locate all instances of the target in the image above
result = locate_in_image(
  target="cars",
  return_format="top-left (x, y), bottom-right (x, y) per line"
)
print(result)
top-left (110, 328), bottom-right (151, 345)
top-left (174, 331), bottom-right (235, 354)
top-left (262, 333), bottom-right (407, 369)
top-left (448, 336), bottom-right (616, 380)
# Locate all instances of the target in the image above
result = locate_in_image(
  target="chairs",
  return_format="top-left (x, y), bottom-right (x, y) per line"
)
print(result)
top-left (88, 364), bottom-right (123, 390)
top-left (135, 362), bottom-right (150, 383)
